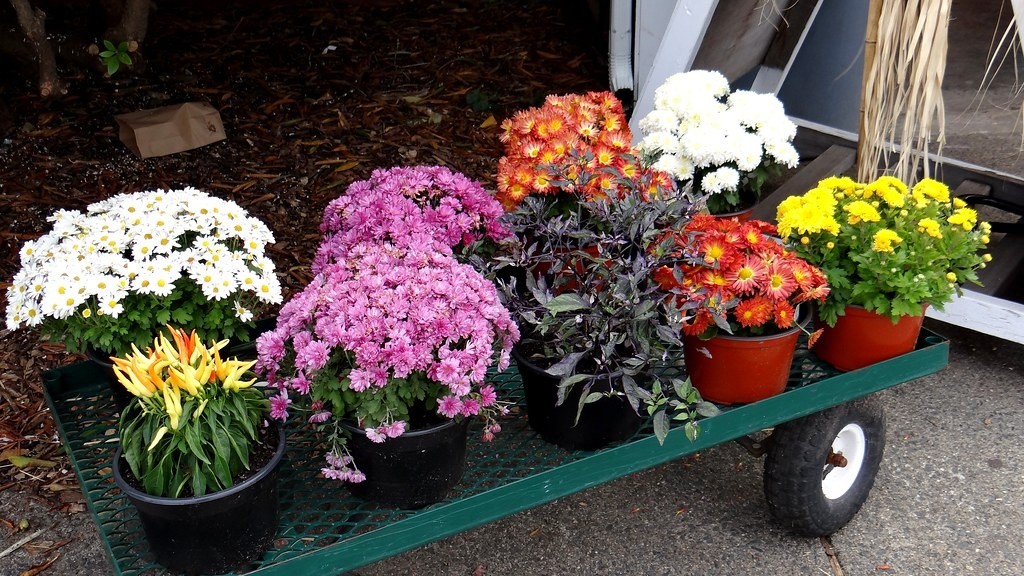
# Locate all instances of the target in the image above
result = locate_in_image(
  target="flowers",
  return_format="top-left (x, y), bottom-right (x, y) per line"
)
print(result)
top-left (4, 68), bottom-right (994, 499)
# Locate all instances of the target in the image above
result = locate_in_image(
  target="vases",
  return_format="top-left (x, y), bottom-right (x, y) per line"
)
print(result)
top-left (336, 411), bottom-right (471, 509)
top-left (679, 300), bottom-right (812, 406)
top-left (812, 299), bottom-right (929, 373)
top-left (111, 411), bottom-right (286, 575)
top-left (713, 206), bottom-right (757, 223)
top-left (513, 323), bottom-right (645, 450)
top-left (91, 345), bottom-right (140, 421)
top-left (535, 244), bottom-right (611, 296)
